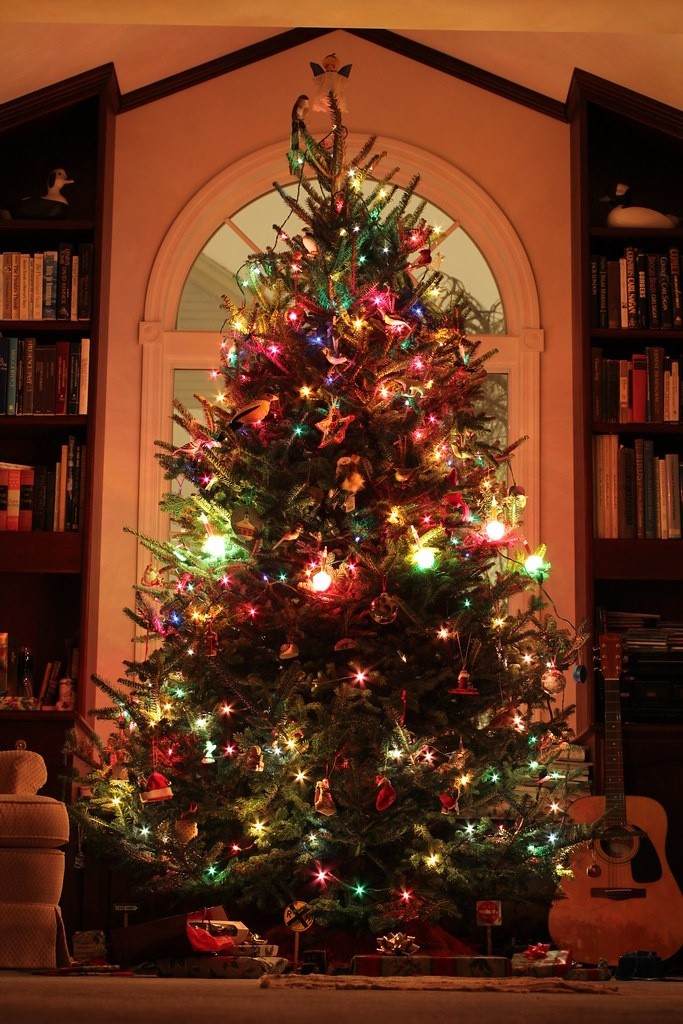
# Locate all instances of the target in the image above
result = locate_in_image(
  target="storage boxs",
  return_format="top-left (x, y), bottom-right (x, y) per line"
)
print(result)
top-left (350, 948), bottom-right (608, 979)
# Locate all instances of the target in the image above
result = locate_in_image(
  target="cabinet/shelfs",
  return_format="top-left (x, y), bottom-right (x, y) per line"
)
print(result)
top-left (566, 68), bottom-right (683, 876)
top-left (0, 63), bottom-right (126, 798)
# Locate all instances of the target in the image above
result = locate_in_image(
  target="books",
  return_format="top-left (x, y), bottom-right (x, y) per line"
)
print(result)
top-left (588, 246), bottom-right (683, 329)
top-left (0, 633), bottom-right (80, 712)
top-left (592, 434), bottom-right (683, 539)
top-left (0, 434), bottom-right (87, 532)
top-left (604, 611), bottom-right (683, 652)
top-left (591, 346), bottom-right (683, 424)
top-left (0, 336), bottom-right (91, 416)
top-left (0, 243), bottom-right (92, 320)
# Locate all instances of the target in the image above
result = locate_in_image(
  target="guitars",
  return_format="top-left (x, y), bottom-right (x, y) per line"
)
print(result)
top-left (546, 632), bottom-right (683, 968)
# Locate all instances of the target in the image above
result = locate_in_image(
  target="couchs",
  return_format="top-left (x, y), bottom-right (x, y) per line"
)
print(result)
top-left (0, 747), bottom-right (70, 975)
